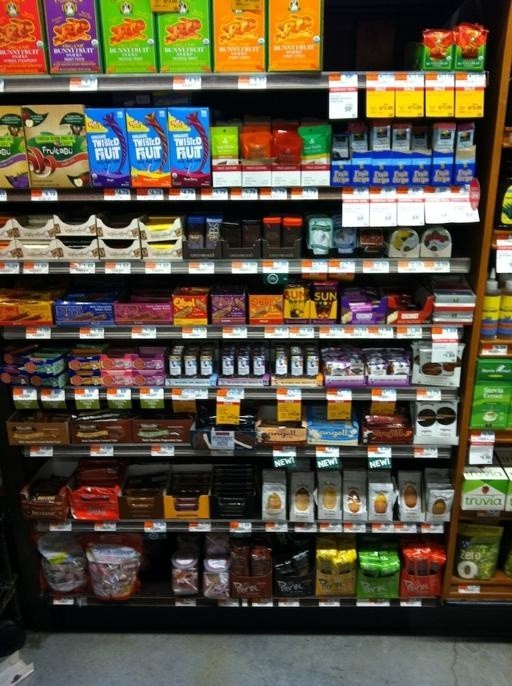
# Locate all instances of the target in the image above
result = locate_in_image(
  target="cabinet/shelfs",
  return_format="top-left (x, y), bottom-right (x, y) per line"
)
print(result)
top-left (1, 65), bottom-right (493, 641)
top-left (437, 1), bottom-right (512, 646)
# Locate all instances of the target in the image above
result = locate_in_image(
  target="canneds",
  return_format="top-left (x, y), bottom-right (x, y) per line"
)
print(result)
top-left (273, 346), bottom-right (319, 378)
top-left (220, 346), bottom-right (264, 376)
top-left (169, 342), bottom-right (215, 380)
